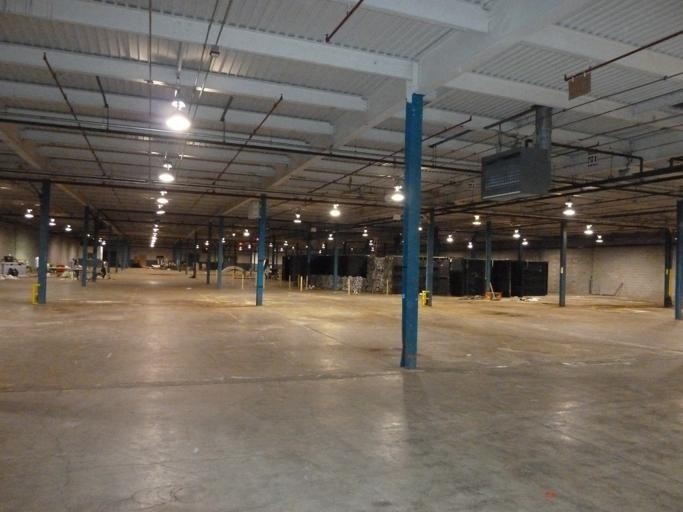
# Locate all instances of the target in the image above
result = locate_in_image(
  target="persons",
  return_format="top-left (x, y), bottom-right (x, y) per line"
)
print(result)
top-left (73, 259), bottom-right (80, 280)
